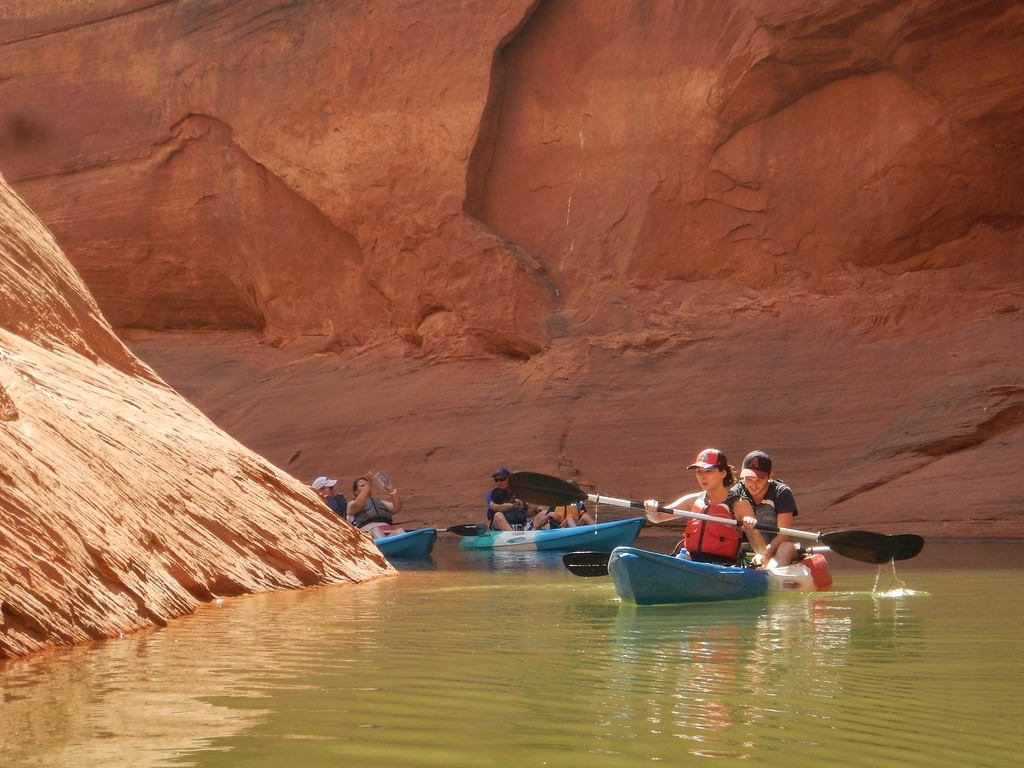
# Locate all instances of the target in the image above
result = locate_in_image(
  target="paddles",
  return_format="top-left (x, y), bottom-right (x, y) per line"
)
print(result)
top-left (490, 487), bottom-right (541, 514)
top-left (477, 523), bottom-right (494, 536)
top-left (364, 523), bottom-right (480, 537)
top-left (507, 471), bottom-right (900, 566)
top-left (561, 532), bottom-right (926, 578)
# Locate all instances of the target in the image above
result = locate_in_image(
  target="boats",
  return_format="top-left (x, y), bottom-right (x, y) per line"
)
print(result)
top-left (606, 545), bottom-right (833, 606)
top-left (373, 527), bottom-right (437, 557)
top-left (459, 515), bottom-right (647, 554)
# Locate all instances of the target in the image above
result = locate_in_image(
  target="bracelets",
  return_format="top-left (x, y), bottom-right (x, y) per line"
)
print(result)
top-left (389, 489), bottom-right (397, 495)
top-left (522, 502), bottom-right (528, 511)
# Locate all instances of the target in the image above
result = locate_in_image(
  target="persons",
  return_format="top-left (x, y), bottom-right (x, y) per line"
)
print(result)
top-left (729, 448), bottom-right (798, 571)
top-left (484, 468), bottom-right (549, 533)
top-left (312, 475), bottom-right (348, 520)
top-left (345, 472), bottom-right (406, 538)
top-left (534, 476), bottom-right (596, 527)
top-left (640, 448), bottom-right (756, 565)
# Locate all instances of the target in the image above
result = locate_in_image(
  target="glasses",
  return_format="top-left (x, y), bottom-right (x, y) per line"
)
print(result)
top-left (494, 477), bottom-right (508, 482)
top-left (323, 486), bottom-right (334, 491)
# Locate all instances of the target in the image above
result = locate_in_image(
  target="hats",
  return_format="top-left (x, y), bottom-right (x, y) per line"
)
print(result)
top-left (740, 450), bottom-right (772, 478)
top-left (312, 477), bottom-right (337, 490)
top-left (686, 448), bottom-right (727, 470)
top-left (491, 468), bottom-right (509, 478)
